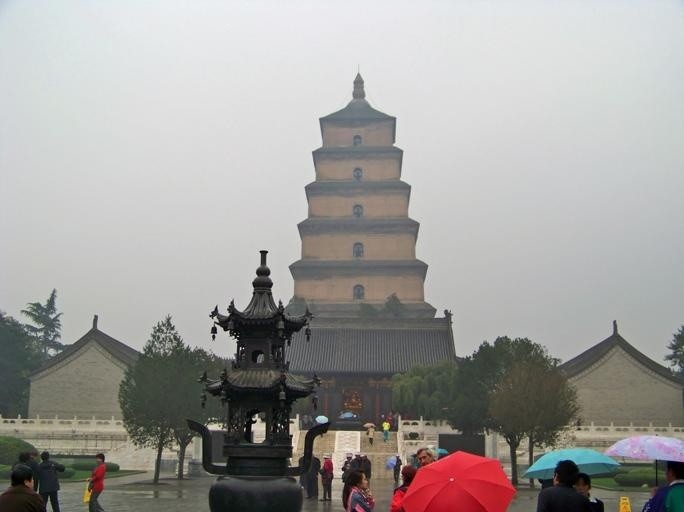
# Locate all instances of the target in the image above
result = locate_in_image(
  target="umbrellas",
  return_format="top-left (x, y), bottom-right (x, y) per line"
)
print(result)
top-left (521, 448), bottom-right (619, 480)
top-left (363, 422), bottom-right (376, 430)
top-left (435, 448), bottom-right (450, 455)
top-left (603, 435), bottom-right (684, 488)
top-left (315, 415), bottom-right (330, 425)
top-left (403, 451), bottom-right (515, 512)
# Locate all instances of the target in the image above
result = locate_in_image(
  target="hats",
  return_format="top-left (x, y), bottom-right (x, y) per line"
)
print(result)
top-left (323, 453), bottom-right (330, 459)
top-left (346, 452), bottom-right (367, 458)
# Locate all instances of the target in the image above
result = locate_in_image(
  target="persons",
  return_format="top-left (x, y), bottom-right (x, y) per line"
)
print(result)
top-left (537, 477), bottom-right (554, 489)
top-left (319, 453), bottom-right (334, 501)
top-left (366, 424), bottom-right (375, 446)
top-left (28, 451), bottom-right (38, 460)
top-left (0, 464), bottom-right (46, 512)
top-left (648, 460), bottom-right (684, 511)
top-left (34, 451), bottom-right (65, 512)
top-left (537, 460), bottom-right (589, 512)
top-left (378, 411), bottom-right (400, 442)
top-left (11, 450), bottom-right (39, 491)
top-left (573, 472), bottom-right (605, 512)
top-left (83, 453), bottom-right (108, 512)
top-left (319, 432), bottom-right (324, 439)
top-left (341, 448), bottom-right (446, 512)
top-left (298, 413), bottom-right (313, 430)
top-left (305, 453), bottom-right (320, 500)
